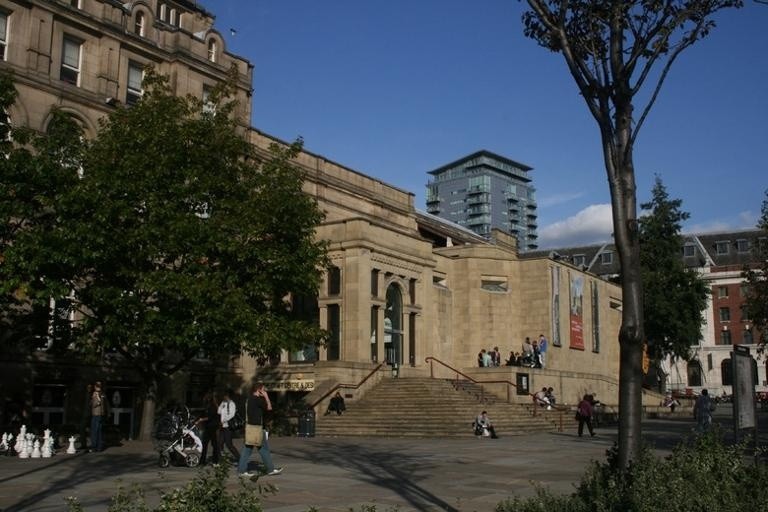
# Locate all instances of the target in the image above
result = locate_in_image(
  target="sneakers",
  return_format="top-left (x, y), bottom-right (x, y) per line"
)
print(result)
top-left (267, 466), bottom-right (283, 476)
top-left (237, 470), bottom-right (254, 477)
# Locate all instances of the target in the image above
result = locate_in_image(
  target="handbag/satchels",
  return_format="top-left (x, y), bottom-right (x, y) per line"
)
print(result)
top-left (244, 422), bottom-right (263, 447)
top-left (228, 417), bottom-right (241, 430)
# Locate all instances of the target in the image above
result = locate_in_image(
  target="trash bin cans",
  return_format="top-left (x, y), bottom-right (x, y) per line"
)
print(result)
top-left (672, 390), bottom-right (680, 399)
top-left (296, 410), bottom-right (315, 437)
top-left (686, 389), bottom-right (692, 397)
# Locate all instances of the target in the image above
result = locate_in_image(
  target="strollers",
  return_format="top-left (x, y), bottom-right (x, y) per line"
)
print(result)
top-left (156, 415), bottom-right (208, 467)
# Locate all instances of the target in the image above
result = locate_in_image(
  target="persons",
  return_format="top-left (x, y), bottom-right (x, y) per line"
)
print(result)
top-left (588, 394), bottom-right (600, 407)
top-left (535, 387), bottom-right (551, 409)
top-left (478, 333), bottom-right (548, 369)
top-left (546, 387), bottom-right (559, 409)
top-left (664, 392), bottom-right (680, 407)
top-left (473, 410), bottom-right (499, 439)
top-left (577, 393), bottom-right (595, 438)
top-left (216, 391), bottom-right (239, 460)
top-left (87, 381), bottom-right (106, 452)
top-left (692, 388), bottom-right (717, 433)
top-left (199, 387), bottom-right (220, 466)
top-left (324, 391), bottom-right (345, 416)
top-left (238, 382), bottom-right (288, 477)
